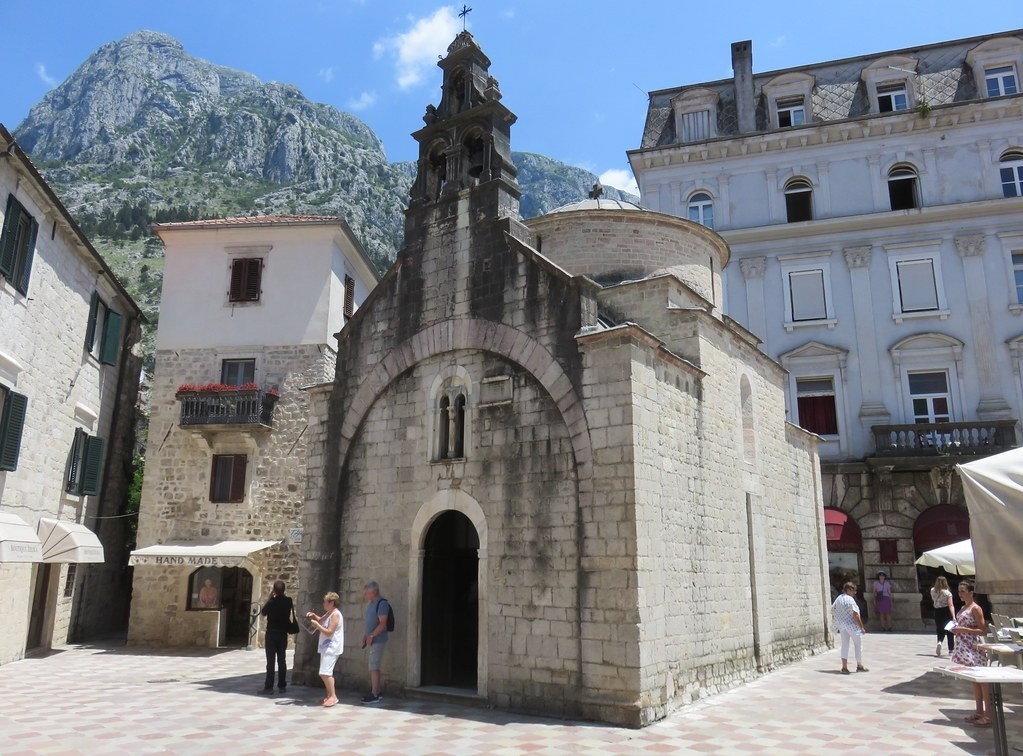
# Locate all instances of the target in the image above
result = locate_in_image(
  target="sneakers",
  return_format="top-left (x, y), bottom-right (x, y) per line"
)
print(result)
top-left (948, 650), bottom-right (954, 655)
top-left (936, 642), bottom-right (941, 656)
top-left (361, 693), bottom-right (383, 704)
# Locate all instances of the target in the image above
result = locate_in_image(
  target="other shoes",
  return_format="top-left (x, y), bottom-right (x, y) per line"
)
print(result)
top-left (841, 668), bottom-right (850, 674)
top-left (882, 627), bottom-right (892, 633)
top-left (278, 689), bottom-right (287, 694)
top-left (315, 697), bottom-right (339, 707)
top-left (856, 665), bottom-right (869, 672)
top-left (258, 690), bottom-right (274, 695)
top-left (964, 712), bottom-right (992, 727)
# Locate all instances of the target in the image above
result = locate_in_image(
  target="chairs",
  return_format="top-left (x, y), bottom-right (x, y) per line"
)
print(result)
top-left (988, 612), bottom-right (1023, 646)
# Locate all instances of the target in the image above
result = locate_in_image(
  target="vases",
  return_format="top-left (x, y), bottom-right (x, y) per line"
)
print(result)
top-left (178, 390), bottom-right (258, 396)
top-left (266, 393), bottom-right (280, 401)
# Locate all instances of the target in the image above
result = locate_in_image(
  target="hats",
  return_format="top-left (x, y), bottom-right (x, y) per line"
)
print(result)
top-left (877, 570), bottom-right (887, 577)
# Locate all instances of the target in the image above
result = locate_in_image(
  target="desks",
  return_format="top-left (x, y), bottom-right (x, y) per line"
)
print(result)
top-left (973, 643), bottom-right (1023, 695)
top-left (933, 666), bottom-right (1023, 756)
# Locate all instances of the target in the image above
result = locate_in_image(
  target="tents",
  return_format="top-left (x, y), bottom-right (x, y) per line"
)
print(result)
top-left (956, 445), bottom-right (1023, 595)
top-left (914, 538), bottom-right (975, 576)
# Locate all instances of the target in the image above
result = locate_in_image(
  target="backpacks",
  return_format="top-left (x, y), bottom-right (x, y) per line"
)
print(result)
top-left (376, 598), bottom-right (395, 632)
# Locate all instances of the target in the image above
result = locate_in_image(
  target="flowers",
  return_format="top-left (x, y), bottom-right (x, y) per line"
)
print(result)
top-left (179, 383), bottom-right (259, 391)
top-left (266, 388), bottom-right (279, 395)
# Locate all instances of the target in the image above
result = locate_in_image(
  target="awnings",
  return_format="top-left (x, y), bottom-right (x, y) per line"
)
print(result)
top-left (128, 540), bottom-right (282, 568)
top-left (0, 513), bottom-right (43, 563)
top-left (823, 508), bottom-right (863, 549)
top-left (37, 517), bottom-right (105, 564)
top-left (914, 503), bottom-right (972, 552)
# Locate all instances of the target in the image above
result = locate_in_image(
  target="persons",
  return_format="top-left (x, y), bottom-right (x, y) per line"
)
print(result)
top-left (949, 580), bottom-right (992, 727)
top-left (930, 576), bottom-right (956, 657)
top-left (199, 579), bottom-right (217, 608)
top-left (258, 581), bottom-right (293, 695)
top-left (873, 571), bottom-right (894, 632)
top-left (305, 592), bottom-right (343, 707)
top-left (833, 582), bottom-right (869, 673)
top-left (360, 581), bottom-right (390, 704)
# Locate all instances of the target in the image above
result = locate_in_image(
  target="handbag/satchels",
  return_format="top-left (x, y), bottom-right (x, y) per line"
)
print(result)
top-left (876, 581), bottom-right (885, 601)
top-left (287, 597), bottom-right (300, 634)
